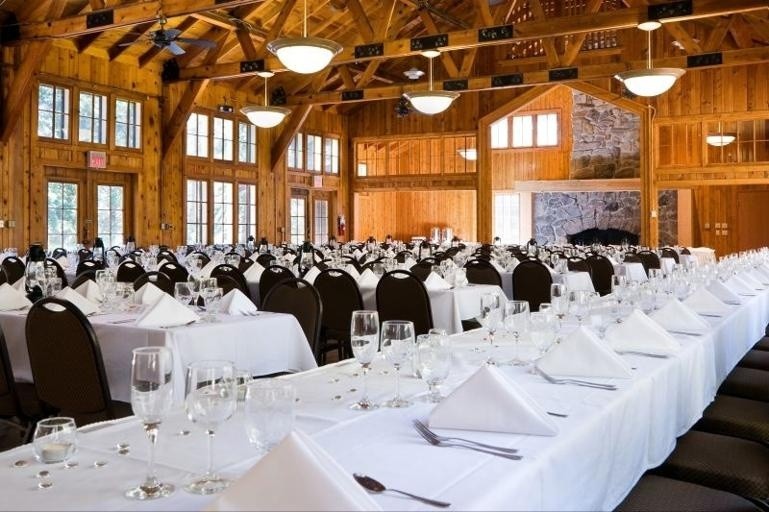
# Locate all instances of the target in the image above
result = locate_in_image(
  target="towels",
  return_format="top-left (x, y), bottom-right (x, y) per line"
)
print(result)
top-left (648, 297), bottom-right (711, 332)
top-left (133, 291), bottom-right (201, 327)
top-left (57, 255), bottom-right (69, 267)
top-left (283, 253), bottom-right (292, 261)
top-left (203, 426), bottom-right (381, 511)
top-left (74, 278), bottom-right (103, 303)
top-left (216, 288), bottom-right (258, 315)
top-left (527, 324), bottom-right (635, 379)
top-left (156, 258), bottom-right (177, 270)
top-left (303, 265), bottom-right (321, 284)
top-left (505, 257), bottom-right (521, 272)
top-left (248, 252), bottom-right (259, 262)
top-left (352, 248), bottom-right (361, 257)
top-left (706, 279), bottom-right (743, 304)
top-left (201, 260), bottom-right (215, 272)
top-left (746, 267), bottom-right (769, 285)
top-left (135, 282), bottom-right (164, 304)
top-left (603, 308), bottom-right (680, 356)
top-left (724, 274), bottom-right (757, 295)
top-left (344, 263), bottom-right (361, 280)
top-left (682, 287), bottom-right (733, 313)
top-left (357, 268), bottom-right (380, 284)
top-left (756, 264), bottom-right (769, 277)
top-left (425, 364), bottom-right (560, 437)
top-left (404, 256), bottom-right (417, 269)
top-left (12, 275), bottom-right (27, 296)
top-left (488, 259), bottom-right (508, 273)
top-left (424, 270), bottom-right (453, 289)
top-left (243, 262), bottom-right (266, 278)
top-left (737, 271), bottom-right (766, 290)
top-left (0, 281), bottom-right (33, 312)
top-left (117, 257), bottom-right (135, 268)
top-left (45, 286), bottom-right (99, 315)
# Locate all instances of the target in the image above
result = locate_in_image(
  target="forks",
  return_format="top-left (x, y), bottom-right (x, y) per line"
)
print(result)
top-left (413, 426), bottom-right (524, 460)
top-left (533, 367), bottom-right (619, 391)
top-left (412, 419), bottom-right (519, 453)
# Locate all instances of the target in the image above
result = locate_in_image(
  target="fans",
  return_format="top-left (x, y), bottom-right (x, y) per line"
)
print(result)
top-left (118, 16), bottom-right (216, 55)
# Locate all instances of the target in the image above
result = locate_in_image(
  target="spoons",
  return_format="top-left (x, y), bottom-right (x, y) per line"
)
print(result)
top-left (353, 472), bottom-right (452, 508)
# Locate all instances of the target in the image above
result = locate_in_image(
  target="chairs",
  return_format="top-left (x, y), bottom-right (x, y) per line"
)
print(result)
top-left (612, 475), bottom-right (766, 512)
top-left (76, 260), bottom-right (101, 277)
top-left (0, 256), bottom-right (25, 290)
top-left (0, 256), bottom-right (26, 284)
top-left (722, 366), bottom-right (769, 405)
top-left (741, 348), bottom-right (769, 371)
top-left (72, 270), bottom-right (96, 290)
top-left (158, 262), bottom-right (195, 284)
top-left (259, 278), bottom-right (323, 355)
top-left (464, 258), bottom-right (502, 288)
top-left (0, 321), bottom-right (33, 420)
top-left (133, 272), bottom-right (173, 296)
top-left (377, 268), bottom-right (433, 339)
top-left (313, 268), bottom-right (367, 360)
top-left (666, 430), bottom-right (769, 499)
top-left (116, 261), bottom-right (147, 282)
top-left (211, 263), bottom-right (249, 294)
top-left (588, 254), bottom-right (620, 297)
top-left (512, 261), bottom-right (553, 311)
top-left (702, 395), bottom-right (769, 445)
top-left (25, 297), bottom-right (116, 446)
top-left (199, 273), bottom-right (240, 307)
top-left (260, 265), bottom-right (294, 307)
top-left (755, 336), bottom-right (768, 351)
top-left (566, 256), bottom-right (586, 270)
top-left (45, 256), bottom-right (70, 288)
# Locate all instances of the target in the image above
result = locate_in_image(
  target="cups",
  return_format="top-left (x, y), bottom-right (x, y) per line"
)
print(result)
top-left (124, 345), bottom-right (177, 500)
top-left (540, 303), bottom-right (550, 314)
top-left (428, 328), bottom-right (446, 347)
top-left (381, 320), bottom-right (415, 409)
top-left (550, 284), bottom-right (568, 332)
top-left (188, 272), bottom-right (202, 310)
top-left (204, 288), bottom-right (222, 312)
top-left (96, 270), bottom-right (114, 307)
top-left (504, 300), bottom-right (530, 367)
top-left (531, 311), bottom-right (545, 333)
top-left (611, 275), bottom-right (627, 294)
top-left (244, 378), bottom-right (295, 454)
top-left (235, 370), bottom-right (253, 402)
top-left (551, 254), bottom-right (558, 268)
top-left (569, 291), bottom-right (577, 314)
top-left (34, 416), bottom-right (77, 464)
top-left (417, 335), bottom-right (449, 403)
top-left (475, 293), bottom-right (502, 367)
top-left (672, 264), bottom-right (685, 275)
top-left (649, 269), bottom-right (661, 285)
top-left (182, 360), bottom-right (237, 496)
top-left (200, 278), bottom-right (216, 299)
top-left (174, 283), bottom-right (193, 305)
top-left (348, 310), bottom-right (380, 411)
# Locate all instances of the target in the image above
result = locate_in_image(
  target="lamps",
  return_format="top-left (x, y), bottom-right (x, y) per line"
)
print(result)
top-left (615, 21), bottom-right (686, 96)
top-left (240, 72), bottom-right (291, 128)
top-left (706, 122), bottom-right (736, 147)
top-left (458, 138), bottom-right (476, 160)
top-left (403, 50), bottom-right (462, 115)
top-left (266, 0), bottom-right (344, 74)
